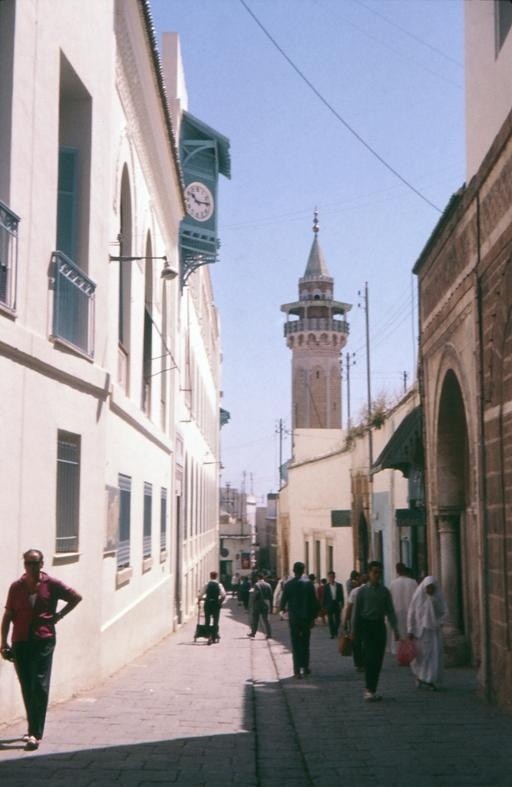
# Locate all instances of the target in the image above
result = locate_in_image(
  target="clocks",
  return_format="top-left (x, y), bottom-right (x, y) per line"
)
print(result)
top-left (182, 181), bottom-right (215, 221)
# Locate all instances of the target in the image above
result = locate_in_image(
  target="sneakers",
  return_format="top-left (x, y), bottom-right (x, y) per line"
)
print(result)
top-left (21, 731), bottom-right (29, 741)
top-left (362, 686), bottom-right (377, 700)
top-left (303, 668), bottom-right (311, 675)
top-left (27, 740), bottom-right (40, 751)
top-left (414, 678), bottom-right (420, 687)
top-left (424, 684), bottom-right (437, 690)
top-left (247, 632), bottom-right (256, 637)
top-left (293, 671), bottom-right (301, 677)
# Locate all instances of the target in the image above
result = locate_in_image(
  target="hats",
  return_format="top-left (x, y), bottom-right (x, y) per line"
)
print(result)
top-left (327, 572), bottom-right (335, 577)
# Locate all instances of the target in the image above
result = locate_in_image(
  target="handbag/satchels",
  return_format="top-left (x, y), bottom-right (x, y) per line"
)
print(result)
top-left (337, 636), bottom-right (353, 656)
top-left (397, 639), bottom-right (415, 665)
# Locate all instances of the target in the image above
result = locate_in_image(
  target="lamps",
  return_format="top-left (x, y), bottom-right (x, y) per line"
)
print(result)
top-left (109, 255), bottom-right (178, 280)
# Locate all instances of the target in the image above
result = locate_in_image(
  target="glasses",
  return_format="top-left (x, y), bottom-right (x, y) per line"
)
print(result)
top-left (23, 558), bottom-right (41, 565)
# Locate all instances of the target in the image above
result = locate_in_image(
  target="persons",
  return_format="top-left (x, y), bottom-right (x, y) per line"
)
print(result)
top-left (199, 571), bottom-right (227, 645)
top-left (230, 562), bottom-right (450, 701)
top-left (0, 550), bottom-right (82, 748)
